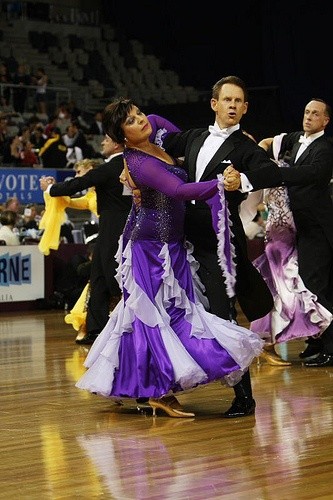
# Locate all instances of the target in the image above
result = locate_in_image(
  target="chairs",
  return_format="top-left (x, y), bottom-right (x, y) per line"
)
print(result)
top-left (1, 29), bottom-right (199, 168)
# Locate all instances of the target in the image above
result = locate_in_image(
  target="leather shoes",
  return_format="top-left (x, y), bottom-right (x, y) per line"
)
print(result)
top-left (222, 396), bottom-right (256, 418)
top-left (75, 333), bottom-right (98, 344)
top-left (299, 345), bottom-right (320, 359)
top-left (304, 352), bottom-right (333, 367)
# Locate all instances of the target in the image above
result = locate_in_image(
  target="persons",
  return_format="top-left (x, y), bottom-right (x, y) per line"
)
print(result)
top-left (38, 136), bottom-right (134, 346)
top-left (236, 98), bottom-right (333, 368)
top-left (74, 76), bottom-right (279, 420)
top-left (0, 62), bottom-right (112, 288)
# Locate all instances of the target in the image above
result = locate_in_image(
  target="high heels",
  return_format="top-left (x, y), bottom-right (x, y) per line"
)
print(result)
top-left (136, 399), bottom-right (152, 415)
top-left (255, 348), bottom-right (292, 366)
top-left (148, 395), bottom-right (196, 418)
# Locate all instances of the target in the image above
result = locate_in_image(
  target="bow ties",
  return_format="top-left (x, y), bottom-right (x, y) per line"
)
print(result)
top-left (208, 124), bottom-right (229, 139)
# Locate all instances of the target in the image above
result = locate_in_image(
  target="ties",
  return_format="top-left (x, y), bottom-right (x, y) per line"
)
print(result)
top-left (299, 134), bottom-right (312, 145)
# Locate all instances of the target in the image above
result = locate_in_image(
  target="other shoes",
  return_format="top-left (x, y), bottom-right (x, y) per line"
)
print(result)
top-left (75, 326), bottom-right (86, 341)
